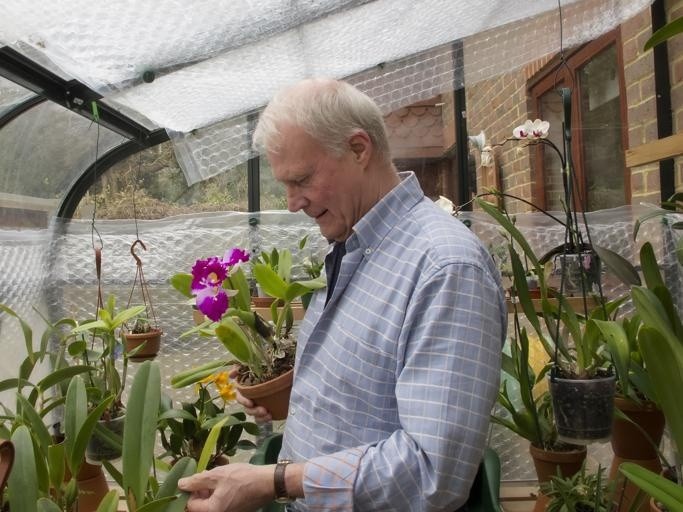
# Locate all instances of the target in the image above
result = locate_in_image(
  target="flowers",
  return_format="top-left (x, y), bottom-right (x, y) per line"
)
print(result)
top-left (467, 116), bottom-right (590, 252)
top-left (171, 244), bottom-right (325, 377)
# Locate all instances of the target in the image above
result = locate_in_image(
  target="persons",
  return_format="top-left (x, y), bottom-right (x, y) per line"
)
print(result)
top-left (177, 75), bottom-right (508, 510)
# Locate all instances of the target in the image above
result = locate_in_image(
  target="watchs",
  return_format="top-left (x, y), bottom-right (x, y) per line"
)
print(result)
top-left (272, 459), bottom-right (296, 504)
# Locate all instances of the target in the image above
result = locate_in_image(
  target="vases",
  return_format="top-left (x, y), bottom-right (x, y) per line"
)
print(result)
top-left (229, 355), bottom-right (296, 421)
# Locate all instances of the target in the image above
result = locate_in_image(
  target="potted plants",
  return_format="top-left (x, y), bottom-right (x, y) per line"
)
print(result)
top-left (475, 194), bottom-right (683, 512)
top-left (120, 317), bottom-right (163, 362)
top-left (250, 247), bottom-right (324, 311)
top-left (71, 294), bottom-right (146, 462)
